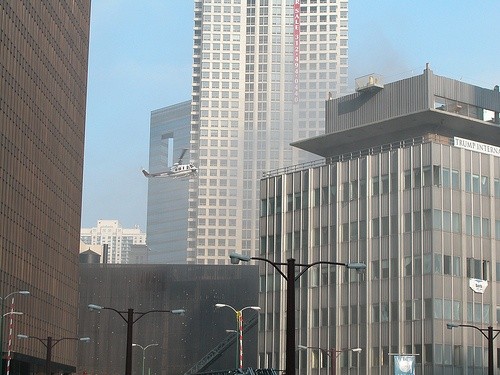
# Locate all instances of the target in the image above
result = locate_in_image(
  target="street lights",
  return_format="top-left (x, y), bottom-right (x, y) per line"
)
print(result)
top-left (87, 303), bottom-right (186, 375)
top-left (446, 323), bottom-right (500, 375)
top-left (0, 290), bottom-right (30, 375)
top-left (230, 252), bottom-right (367, 375)
top-left (132, 344), bottom-right (159, 375)
top-left (296, 345), bottom-right (362, 375)
top-left (16, 334), bottom-right (91, 375)
top-left (215, 303), bottom-right (262, 369)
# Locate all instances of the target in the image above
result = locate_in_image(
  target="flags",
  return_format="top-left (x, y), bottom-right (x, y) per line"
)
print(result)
top-left (393, 356), bottom-right (416, 375)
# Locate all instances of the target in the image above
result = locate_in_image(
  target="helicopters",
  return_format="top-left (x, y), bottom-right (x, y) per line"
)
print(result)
top-left (142, 149), bottom-right (199, 183)
